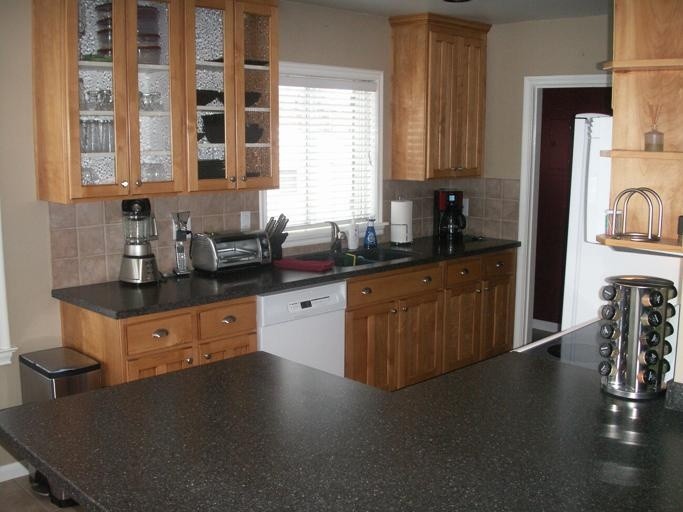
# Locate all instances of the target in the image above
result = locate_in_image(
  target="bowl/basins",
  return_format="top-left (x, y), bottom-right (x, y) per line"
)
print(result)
top-left (200, 113), bottom-right (225, 143)
top-left (197, 89), bottom-right (218, 106)
top-left (216, 91), bottom-right (262, 107)
top-left (245, 123), bottom-right (264, 143)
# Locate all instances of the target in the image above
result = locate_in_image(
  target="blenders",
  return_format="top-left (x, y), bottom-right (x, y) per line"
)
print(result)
top-left (118, 197), bottom-right (168, 284)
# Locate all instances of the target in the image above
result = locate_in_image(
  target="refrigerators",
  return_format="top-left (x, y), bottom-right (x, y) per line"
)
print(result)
top-left (560, 112), bottom-right (681, 332)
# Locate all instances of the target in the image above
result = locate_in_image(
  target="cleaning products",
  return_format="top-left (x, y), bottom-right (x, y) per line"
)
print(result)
top-left (348, 212), bottom-right (359, 250)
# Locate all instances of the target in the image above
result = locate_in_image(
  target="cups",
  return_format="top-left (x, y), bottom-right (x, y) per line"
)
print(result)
top-left (80, 120), bottom-right (115, 153)
top-left (139, 90), bottom-right (164, 111)
top-left (79, 77), bottom-right (113, 111)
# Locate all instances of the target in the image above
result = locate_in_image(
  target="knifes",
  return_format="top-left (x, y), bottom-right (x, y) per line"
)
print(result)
top-left (265, 213), bottom-right (289, 234)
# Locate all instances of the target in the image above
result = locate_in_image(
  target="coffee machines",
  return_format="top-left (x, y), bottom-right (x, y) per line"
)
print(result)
top-left (433, 188), bottom-right (466, 242)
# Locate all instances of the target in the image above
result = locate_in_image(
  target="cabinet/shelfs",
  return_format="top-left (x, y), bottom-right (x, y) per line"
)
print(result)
top-left (59, 296), bottom-right (258, 387)
top-left (343, 246), bottom-right (517, 391)
top-left (595, 1), bottom-right (683, 257)
top-left (388, 12), bottom-right (492, 184)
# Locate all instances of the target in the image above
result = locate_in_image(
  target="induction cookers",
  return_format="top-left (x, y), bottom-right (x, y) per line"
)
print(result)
top-left (522, 319), bottom-right (600, 373)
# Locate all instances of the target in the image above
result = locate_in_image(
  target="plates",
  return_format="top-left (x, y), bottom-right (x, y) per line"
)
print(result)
top-left (198, 159), bottom-right (225, 180)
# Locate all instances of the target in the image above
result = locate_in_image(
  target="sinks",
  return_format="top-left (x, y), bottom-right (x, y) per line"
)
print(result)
top-left (300, 252), bottom-right (376, 268)
top-left (349, 248), bottom-right (422, 262)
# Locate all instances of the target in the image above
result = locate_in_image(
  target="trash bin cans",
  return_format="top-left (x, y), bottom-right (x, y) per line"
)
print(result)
top-left (20, 346), bottom-right (101, 507)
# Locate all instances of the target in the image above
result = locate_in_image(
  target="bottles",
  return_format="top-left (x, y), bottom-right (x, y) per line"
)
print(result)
top-left (605, 210), bottom-right (622, 237)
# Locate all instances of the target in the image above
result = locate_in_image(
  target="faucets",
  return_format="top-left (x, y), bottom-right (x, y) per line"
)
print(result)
top-left (326, 222), bottom-right (347, 255)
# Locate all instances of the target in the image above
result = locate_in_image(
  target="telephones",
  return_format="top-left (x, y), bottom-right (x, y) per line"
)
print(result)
top-left (173, 241), bottom-right (191, 277)
